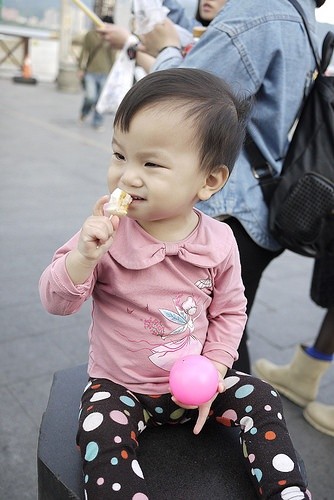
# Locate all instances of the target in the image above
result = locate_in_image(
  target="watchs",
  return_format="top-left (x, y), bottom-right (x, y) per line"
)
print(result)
top-left (127, 40), bottom-right (140, 60)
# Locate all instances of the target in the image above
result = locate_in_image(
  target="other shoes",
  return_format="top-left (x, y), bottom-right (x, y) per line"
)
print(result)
top-left (80, 113), bottom-right (87, 122)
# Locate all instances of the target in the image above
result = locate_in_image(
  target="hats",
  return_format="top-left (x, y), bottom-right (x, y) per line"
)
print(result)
top-left (103, 16), bottom-right (114, 23)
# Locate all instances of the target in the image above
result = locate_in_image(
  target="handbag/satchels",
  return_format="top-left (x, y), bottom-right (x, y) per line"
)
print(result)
top-left (95, 34), bottom-right (139, 116)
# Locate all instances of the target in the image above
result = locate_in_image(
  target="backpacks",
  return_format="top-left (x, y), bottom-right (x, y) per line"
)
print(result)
top-left (229, 0), bottom-right (333, 257)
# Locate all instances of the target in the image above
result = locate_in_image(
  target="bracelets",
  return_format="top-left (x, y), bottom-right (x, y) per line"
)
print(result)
top-left (157, 46), bottom-right (183, 55)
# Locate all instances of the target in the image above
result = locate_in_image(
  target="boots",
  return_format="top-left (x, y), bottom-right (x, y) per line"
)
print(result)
top-left (255, 343), bottom-right (333, 408)
top-left (302, 402), bottom-right (334, 437)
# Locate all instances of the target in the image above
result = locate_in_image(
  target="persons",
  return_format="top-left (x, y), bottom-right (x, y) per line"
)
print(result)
top-left (78, 16), bottom-right (117, 128)
top-left (132, 0), bottom-right (327, 374)
top-left (38, 67), bottom-right (312, 500)
top-left (94, 0), bottom-right (229, 73)
top-left (252, 259), bottom-right (334, 435)
